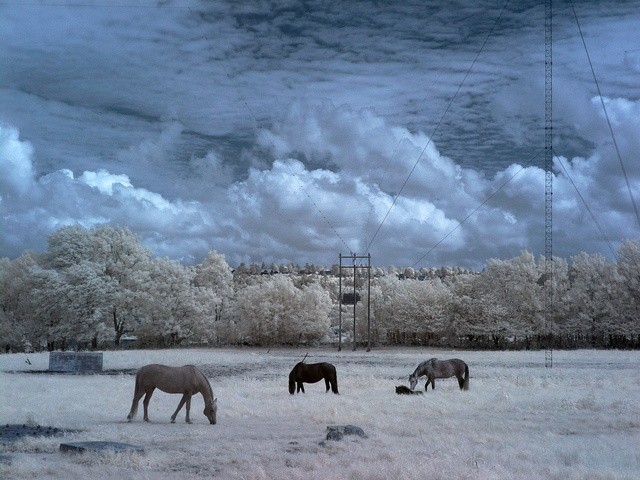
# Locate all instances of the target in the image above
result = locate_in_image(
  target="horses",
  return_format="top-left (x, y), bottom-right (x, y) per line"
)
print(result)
top-left (289, 362), bottom-right (338, 395)
top-left (127, 364), bottom-right (218, 425)
top-left (408, 358), bottom-right (469, 391)
